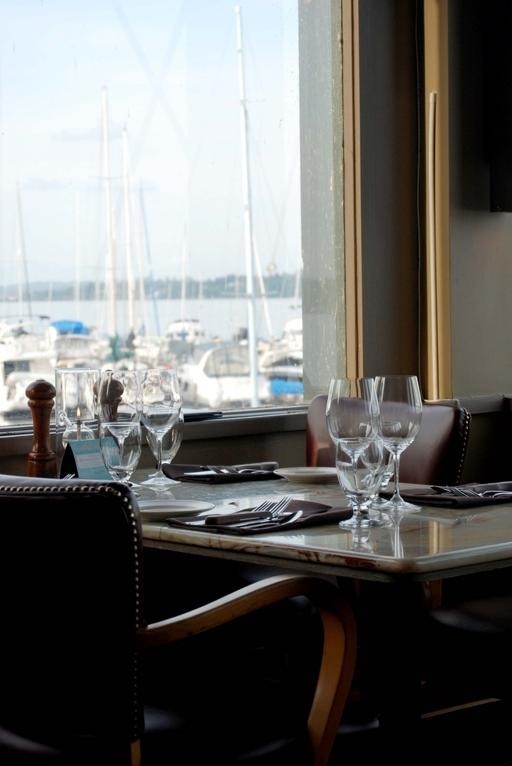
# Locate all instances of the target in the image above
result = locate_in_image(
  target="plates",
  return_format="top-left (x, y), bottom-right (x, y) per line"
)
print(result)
top-left (135, 500), bottom-right (215, 523)
top-left (273, 465), bottom-right (338, 483)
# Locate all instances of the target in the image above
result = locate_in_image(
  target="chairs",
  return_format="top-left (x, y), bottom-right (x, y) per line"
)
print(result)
top-left (304, 394), bottom-right (471, 487)
top-left (0, 476), bottom-right (361, 765)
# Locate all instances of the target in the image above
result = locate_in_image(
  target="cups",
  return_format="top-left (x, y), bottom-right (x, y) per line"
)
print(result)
top-left (53, 367), bottom-right (98, 473)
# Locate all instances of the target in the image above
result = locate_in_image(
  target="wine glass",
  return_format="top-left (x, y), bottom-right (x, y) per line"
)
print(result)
top-left (325, 369), bottom-right (424, 532)
top-left (99, 367), bottom-right (186, 498)
top-left (347, 523), bottom-right (407, 558)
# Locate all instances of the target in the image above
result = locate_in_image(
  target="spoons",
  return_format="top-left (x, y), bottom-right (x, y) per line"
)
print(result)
top-left (260, 508), bottom-right (305, 529)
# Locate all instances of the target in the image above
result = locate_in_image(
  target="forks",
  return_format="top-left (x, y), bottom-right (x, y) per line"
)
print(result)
top-left (207, 464), bottom-right (269, 475)
top-left (451, 486), bottom-right (509, 500)
top-left (222, 493), bottom-right (293, 531)
